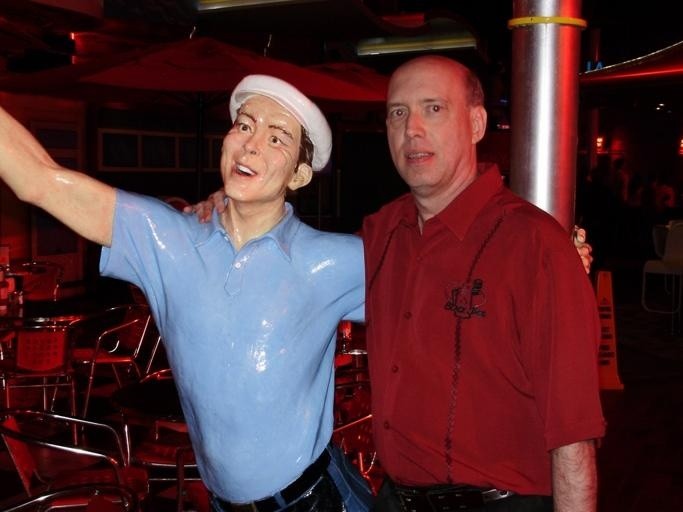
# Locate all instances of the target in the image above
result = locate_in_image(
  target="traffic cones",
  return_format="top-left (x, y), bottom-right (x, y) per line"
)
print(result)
top-left (592, 268), bottom-right (627, 392)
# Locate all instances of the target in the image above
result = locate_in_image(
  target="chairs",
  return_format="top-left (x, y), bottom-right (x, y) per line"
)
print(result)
top-left (642, 219), bottom-right (683, 316)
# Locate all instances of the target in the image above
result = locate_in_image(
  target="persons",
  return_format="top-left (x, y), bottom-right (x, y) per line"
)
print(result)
top-left (0, 75), bottom-right (598, 512)
top-left (184, 55), bottom-right (607, 512)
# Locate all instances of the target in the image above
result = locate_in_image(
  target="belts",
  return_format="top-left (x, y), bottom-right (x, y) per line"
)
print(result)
top-left (380, 479), bottom-right (521, 510)
